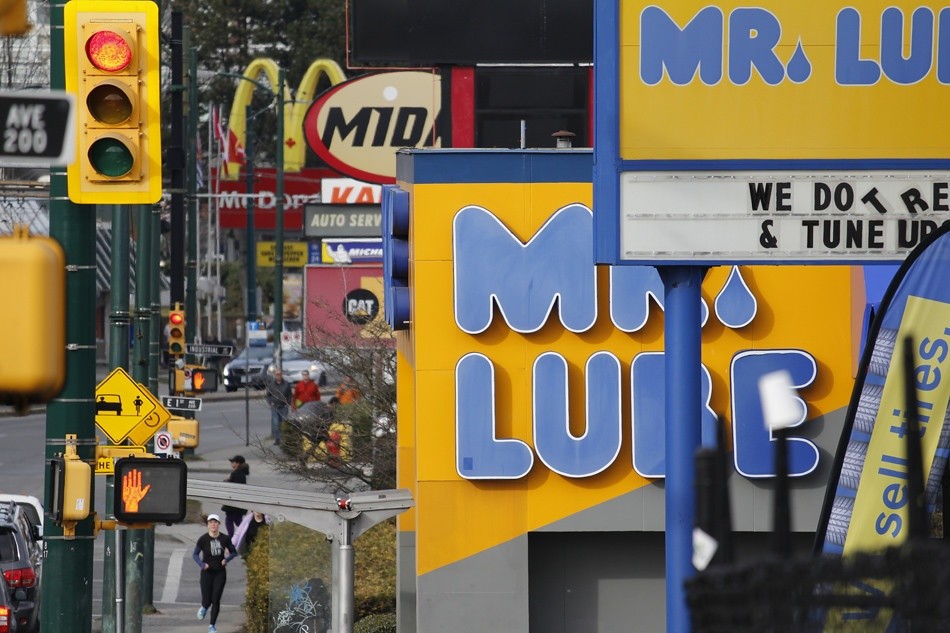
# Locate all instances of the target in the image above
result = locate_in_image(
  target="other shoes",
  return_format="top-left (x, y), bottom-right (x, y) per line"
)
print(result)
top-left (208, 624), bottom-right (218, 633)
top-left (198, 605), bottom-right (207, 620)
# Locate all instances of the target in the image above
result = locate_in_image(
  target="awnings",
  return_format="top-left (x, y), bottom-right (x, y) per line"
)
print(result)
top-left (96, 227), bottom-right (173, 295)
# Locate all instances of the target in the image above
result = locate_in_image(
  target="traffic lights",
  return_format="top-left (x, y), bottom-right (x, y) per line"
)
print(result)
top-left (112, 455), bottom-right (188, 524)
top-left (64, 1), bottom-right (164, 205)
top-left (169, 309), bottom-right (185, 353)
top-left (168, 368), bottom-right (185, 390)
top-left (192, 368), bottom-right (219, 393)
top-left (49, 459), bottom-right (91, 521)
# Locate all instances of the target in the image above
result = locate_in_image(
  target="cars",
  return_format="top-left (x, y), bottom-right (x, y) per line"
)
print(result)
top-left (223, 342), bottom-right (278, 393)
top-left (266, 347), bottom-right (330, 387)
top-left (281, 319), bottom-right (306, 336)
top-left (0, 492), bottom-right (44, 633)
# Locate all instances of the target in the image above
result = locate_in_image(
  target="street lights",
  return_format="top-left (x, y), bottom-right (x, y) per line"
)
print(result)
top-left (187, 68), bottom-right (283, 357)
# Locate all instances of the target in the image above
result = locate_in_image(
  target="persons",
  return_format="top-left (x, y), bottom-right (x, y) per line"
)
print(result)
top-left (221, 455), bottom-right (249, 538)
top-left (193, 514), bottom-right (237, 633)
top-left (226, 509), bottom-right (276, 560)
top-left (326, 397), bottom-right (342, 407)
top-left (337, 375), bottom-right (362, 404)
top-left (292, 370), bottom-right (321, 413)
top-left (265, 367), bottom-right (291, 445)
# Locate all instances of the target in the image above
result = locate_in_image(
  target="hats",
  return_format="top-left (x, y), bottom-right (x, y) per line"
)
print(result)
top-left (229, 456), bottom-right (245, 464)
top-left (207, 514), bottom-right (220, 522)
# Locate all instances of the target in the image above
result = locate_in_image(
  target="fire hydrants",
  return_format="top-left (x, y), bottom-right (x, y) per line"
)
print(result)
top-left (326, 430), bottom-right (342, 467)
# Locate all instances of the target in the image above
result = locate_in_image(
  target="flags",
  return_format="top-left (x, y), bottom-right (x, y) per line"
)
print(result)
top-left (213, 104), bottom-right (223, 139)
top-left (194, 130), bottom-right (203, 192)
top-left (219, 128), bottom-right (229, 161)
top-left (811, 223), bottom-right (950, 633)
top-left (226, 129), bottom-right (246, 165)
